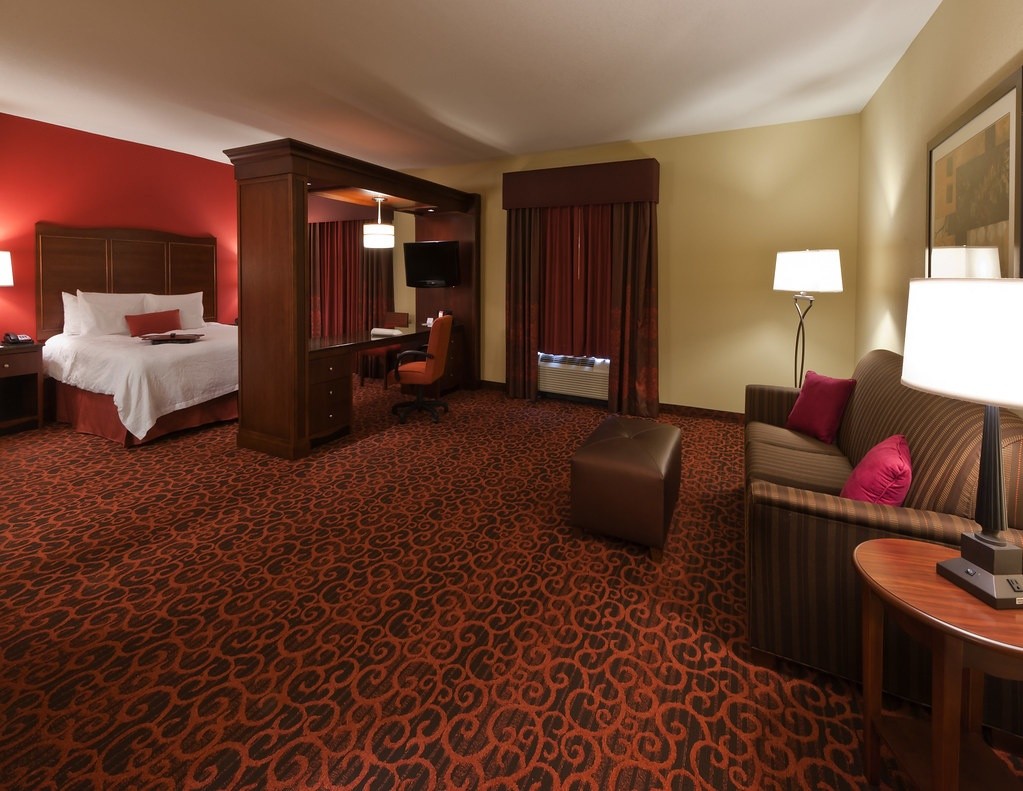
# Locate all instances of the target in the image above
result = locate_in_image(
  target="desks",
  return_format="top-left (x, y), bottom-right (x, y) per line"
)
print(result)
top-left (309, 320), bottom-right (461, 448)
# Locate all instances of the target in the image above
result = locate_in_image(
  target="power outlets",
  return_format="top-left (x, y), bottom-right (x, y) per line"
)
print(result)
top-left (1007, 578), bottom-right (1023, 592)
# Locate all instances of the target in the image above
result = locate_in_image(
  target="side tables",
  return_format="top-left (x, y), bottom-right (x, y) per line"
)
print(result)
top-left (853, 536), bottom-right (1023, 791)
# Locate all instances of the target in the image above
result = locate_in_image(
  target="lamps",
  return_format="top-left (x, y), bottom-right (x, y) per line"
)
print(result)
top-left (924, 245), bottom-right (1002, 279)
top-left (362, 196), bottom-right (395, 249)
top-left (773, 248), bottom-right (844, 388)
top-left (0, 250), bottom-right (14, 287)
top-left (900, 276), bottom-right (1023, 610)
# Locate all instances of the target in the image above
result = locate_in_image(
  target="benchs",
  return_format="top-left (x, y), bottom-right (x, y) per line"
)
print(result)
top-left (571, 416), bottom-right (682, 563)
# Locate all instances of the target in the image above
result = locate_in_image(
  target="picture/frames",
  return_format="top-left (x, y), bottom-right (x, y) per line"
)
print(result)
top-left (925, 65), bottom-right (1023, 279)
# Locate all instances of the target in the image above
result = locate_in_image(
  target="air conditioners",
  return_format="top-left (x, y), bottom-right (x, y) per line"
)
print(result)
top-left (537, 354), bottom-right (610, 401)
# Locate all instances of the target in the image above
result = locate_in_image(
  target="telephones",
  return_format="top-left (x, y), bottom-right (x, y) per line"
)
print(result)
top-left (3, 332), bottom-right (34, 345)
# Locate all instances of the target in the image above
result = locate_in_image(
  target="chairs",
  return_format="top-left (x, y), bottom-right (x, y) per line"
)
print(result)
top-left (386, 315), bottom-right (452, 424)
top-left (360, 312), bottom-right (410, 387)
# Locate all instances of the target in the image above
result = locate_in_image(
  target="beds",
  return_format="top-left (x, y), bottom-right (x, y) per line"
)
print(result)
top-left (35, 225), bottom-right (238, 447)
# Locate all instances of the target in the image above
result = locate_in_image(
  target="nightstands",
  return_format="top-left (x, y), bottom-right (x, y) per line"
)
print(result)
top-left (0, 338), bottom-right (45, 430)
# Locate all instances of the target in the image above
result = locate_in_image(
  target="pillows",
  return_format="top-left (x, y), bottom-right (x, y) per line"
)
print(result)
top-left (141, 291), bottom-right (205, 329)
top-left (76, 289), bottom-right (151, 300)
top-left (783, 371), bottom-right (858, 444)
top-left (125, 309), bottom-right (182, 337)
top-left (839, 435), bottom-right (912, 507)
top-left (61, 291), bottom-right (137, 337)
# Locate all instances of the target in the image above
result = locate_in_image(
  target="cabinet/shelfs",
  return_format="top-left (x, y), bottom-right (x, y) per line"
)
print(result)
top-left (309, 353), bottom-right (352, 443)
top-left (400, 326), bottom-right (462, 396)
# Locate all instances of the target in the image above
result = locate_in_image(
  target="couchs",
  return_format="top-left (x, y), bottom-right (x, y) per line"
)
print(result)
top-left (744, 349), bottom-right (1023, 738)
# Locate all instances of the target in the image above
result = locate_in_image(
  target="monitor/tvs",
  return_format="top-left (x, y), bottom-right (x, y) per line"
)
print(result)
top-left (404, 240), bottom-right (461, 289)
